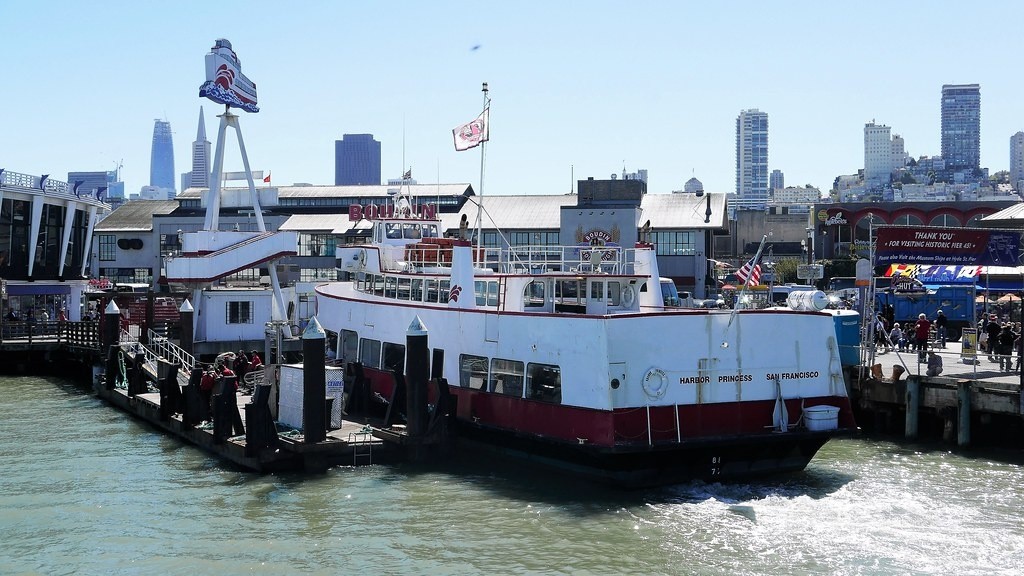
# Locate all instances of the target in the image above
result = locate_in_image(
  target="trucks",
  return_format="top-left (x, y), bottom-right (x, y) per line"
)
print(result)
top-left (861, 284), bottom-right (977, 343)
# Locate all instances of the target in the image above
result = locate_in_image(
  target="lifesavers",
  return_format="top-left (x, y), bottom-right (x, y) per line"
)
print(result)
top-left (620, 285), bottom-right (635, 308)
top-left (643, 368), bottom-right (668, 398)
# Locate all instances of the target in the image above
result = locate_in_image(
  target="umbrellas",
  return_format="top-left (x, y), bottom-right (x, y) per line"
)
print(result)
top-left (975, 296), bottom-right (994, 303)
top-left (996, 292), bottom-right (1021, 308)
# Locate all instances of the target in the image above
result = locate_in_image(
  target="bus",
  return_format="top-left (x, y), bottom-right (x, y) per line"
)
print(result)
top-left (826, 276), bottom-right (914, 307)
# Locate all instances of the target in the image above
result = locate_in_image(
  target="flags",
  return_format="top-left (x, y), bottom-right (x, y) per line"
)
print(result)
top-left (263, 175), bottom-right (270, 182)
top-left (404, 170), bottom-right (411, 179)
top-left (452, 107), bottom-right (489, 151)
top-left (735, 251), bottom-right (762, 287)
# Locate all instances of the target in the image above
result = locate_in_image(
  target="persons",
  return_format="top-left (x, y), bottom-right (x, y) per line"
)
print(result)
top-left (232, 349), bottom-right (248, 386)
top-left (81, 310), bottom-right (100, 322)
top-left (247, 350), bottom-right (262, 392)
top-left (866, 312), bottom-right (918, 359)
top-left (925, 350), bottom-right (943, 376)
top-left (977, 312), bottom-right (1021, 372)
top-left (936, 310), bottom-right (947, 349)
top-left (40, 308), bottom-right (50, 339)
top-left (25, 314), bottom-right (38, 322)
top-left (5, 308), bottom-right (25, 321)
top-left (59, 309), bottom-right (68, 336)
top-left (913, 312), bottom-right (931, 364)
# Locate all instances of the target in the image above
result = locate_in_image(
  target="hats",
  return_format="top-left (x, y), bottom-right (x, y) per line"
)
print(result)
top-left (250, 350), bottom-right (258, 354)
top-left (989, 314), bottom-right (996, 319)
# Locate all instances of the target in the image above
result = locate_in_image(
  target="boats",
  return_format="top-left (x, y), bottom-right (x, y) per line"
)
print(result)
top-left (313, 80), bottom-right (851, 483)
